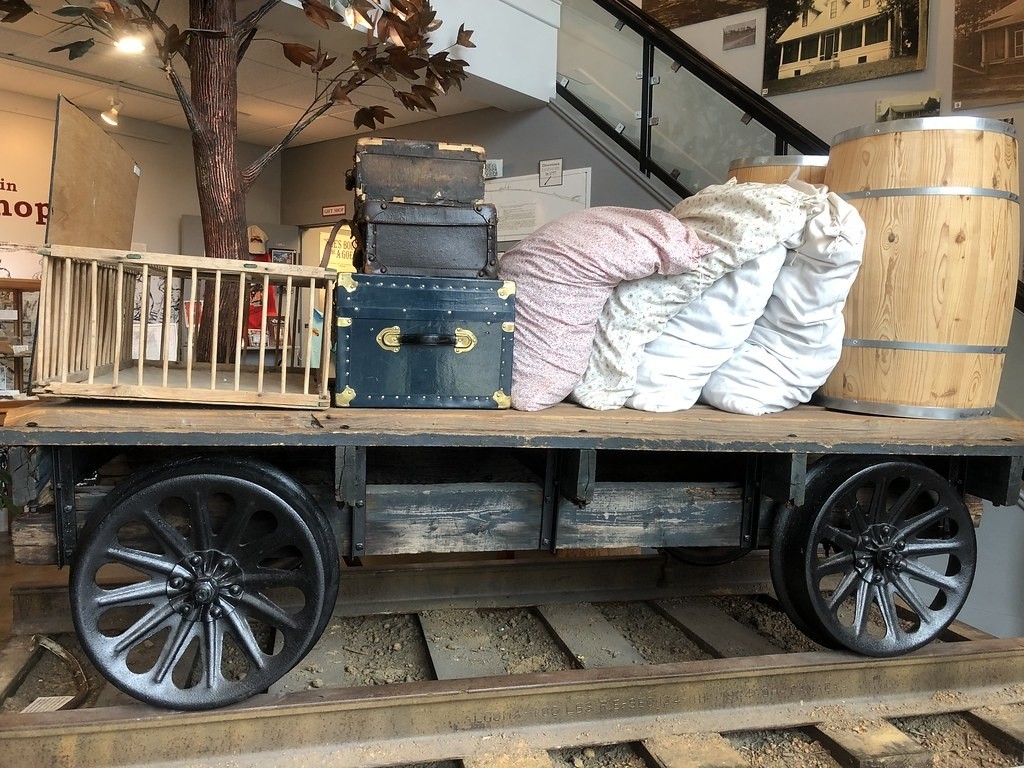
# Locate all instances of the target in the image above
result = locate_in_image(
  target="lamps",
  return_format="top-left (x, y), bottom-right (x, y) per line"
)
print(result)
top-left (99, 96), bottom-right (125, 127)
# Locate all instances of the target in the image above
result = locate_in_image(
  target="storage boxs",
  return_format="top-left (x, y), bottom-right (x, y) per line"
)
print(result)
top-left (345, 136), bottom-right (487, 204)
top-left (349, 202), bottom-right (499, 280)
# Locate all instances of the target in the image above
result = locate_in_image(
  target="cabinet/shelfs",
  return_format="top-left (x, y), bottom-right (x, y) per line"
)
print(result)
top-left (0, 278), bottom-right (41, 426)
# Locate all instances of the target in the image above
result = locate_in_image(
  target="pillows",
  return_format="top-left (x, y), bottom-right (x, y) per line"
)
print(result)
top-left (499, 207), bottom-right (721, 416)
top-left (631, 164), bottom-right (829, 412)
top-left (699, 184), bottom-right (866, 418)
top-left (572, 176), bottom-right (829, 411)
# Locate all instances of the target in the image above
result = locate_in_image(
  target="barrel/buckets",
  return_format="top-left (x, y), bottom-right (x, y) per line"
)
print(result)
top-left (728, 155), bottom-right (829, 186)
top-left (821, 116), bottom-right (1019, 419)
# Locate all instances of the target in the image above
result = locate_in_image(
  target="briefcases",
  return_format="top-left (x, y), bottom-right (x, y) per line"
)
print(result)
top-left (337, 271), bottom-right (516, 409)
top-left (348, 200), bottom-right (499, 279)
top-left (345, 136), bottom-right (487, 204)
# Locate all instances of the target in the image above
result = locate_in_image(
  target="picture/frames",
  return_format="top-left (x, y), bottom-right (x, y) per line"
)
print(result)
top-left (762, 0), bottom-right (928, 95)
